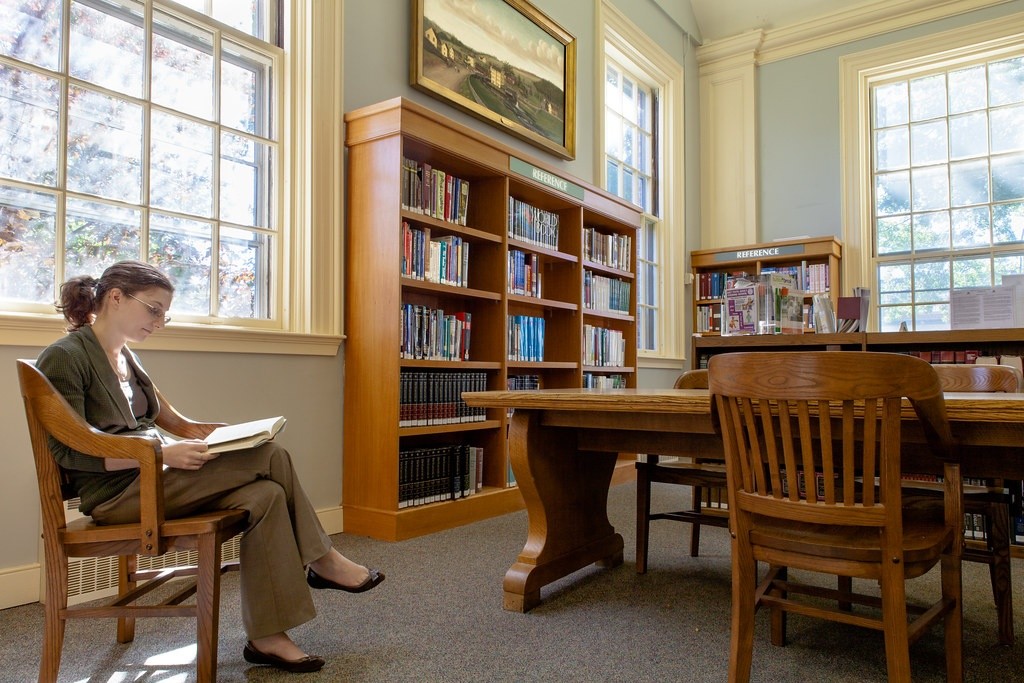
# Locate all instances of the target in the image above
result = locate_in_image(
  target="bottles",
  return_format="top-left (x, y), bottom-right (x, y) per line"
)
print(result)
top-left (755, 275), bottom-right (776, 335)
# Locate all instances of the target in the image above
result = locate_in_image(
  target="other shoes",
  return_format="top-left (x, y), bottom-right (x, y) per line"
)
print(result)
top-left (306, 566), bottom-right (386, 593)
top-left (243, 640), bottom-right (325, 672)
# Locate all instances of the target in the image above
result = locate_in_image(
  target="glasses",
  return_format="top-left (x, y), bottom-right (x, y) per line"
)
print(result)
top-left (129, 294), bottom-right (171, 324)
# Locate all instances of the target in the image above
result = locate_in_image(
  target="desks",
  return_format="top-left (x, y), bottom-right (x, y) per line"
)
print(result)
top-left (461, 387), bottom-right (1024, 613)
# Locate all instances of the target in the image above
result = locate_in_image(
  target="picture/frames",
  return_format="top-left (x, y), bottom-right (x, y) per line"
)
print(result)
top-left (410, 0), bottom-right (579, 161)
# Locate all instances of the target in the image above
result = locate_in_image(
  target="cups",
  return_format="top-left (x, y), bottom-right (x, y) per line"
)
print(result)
top-left (780, 294), bottom-right (804, 334)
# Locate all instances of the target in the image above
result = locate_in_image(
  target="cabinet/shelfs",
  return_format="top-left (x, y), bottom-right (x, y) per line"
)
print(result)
top-left (688, 326), bottom-right (1024, 504)
top-left (342, 96), bottom-right (643, 544)
top-left (691, 235), bottom-right (841, 336)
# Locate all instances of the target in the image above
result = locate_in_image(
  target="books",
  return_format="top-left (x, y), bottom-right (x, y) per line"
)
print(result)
top-left (699, 359), bottom-right (708, 369)
top-left (694, 263), bottom-right (872, 338)
top-left (900, 473), bottom-right (1024, 546)
top-left (397, 144), bottom-right (633, 509)
top-left (880, 348), bottom-right (1023, 392)
top-left (777, 468), bottom-right (844, 503)
top-left (697, 460), bottom-right (728, 509)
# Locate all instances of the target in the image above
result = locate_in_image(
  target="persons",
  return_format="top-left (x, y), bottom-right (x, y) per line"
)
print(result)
top-left (32, 258), bottom-right (387, 673)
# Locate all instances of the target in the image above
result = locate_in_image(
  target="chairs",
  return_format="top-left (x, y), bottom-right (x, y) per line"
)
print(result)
top-left (16, 358), bottom-right (251, 683)
top-left (634, 350), bottom-right (1022, 683)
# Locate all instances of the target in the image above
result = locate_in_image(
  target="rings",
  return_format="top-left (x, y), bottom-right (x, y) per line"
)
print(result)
top-left (199, 453), bottom-right (204, 460)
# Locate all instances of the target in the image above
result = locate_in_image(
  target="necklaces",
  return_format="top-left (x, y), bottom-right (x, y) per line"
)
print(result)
top-left (103, 338), bottom-right (126, 378)
